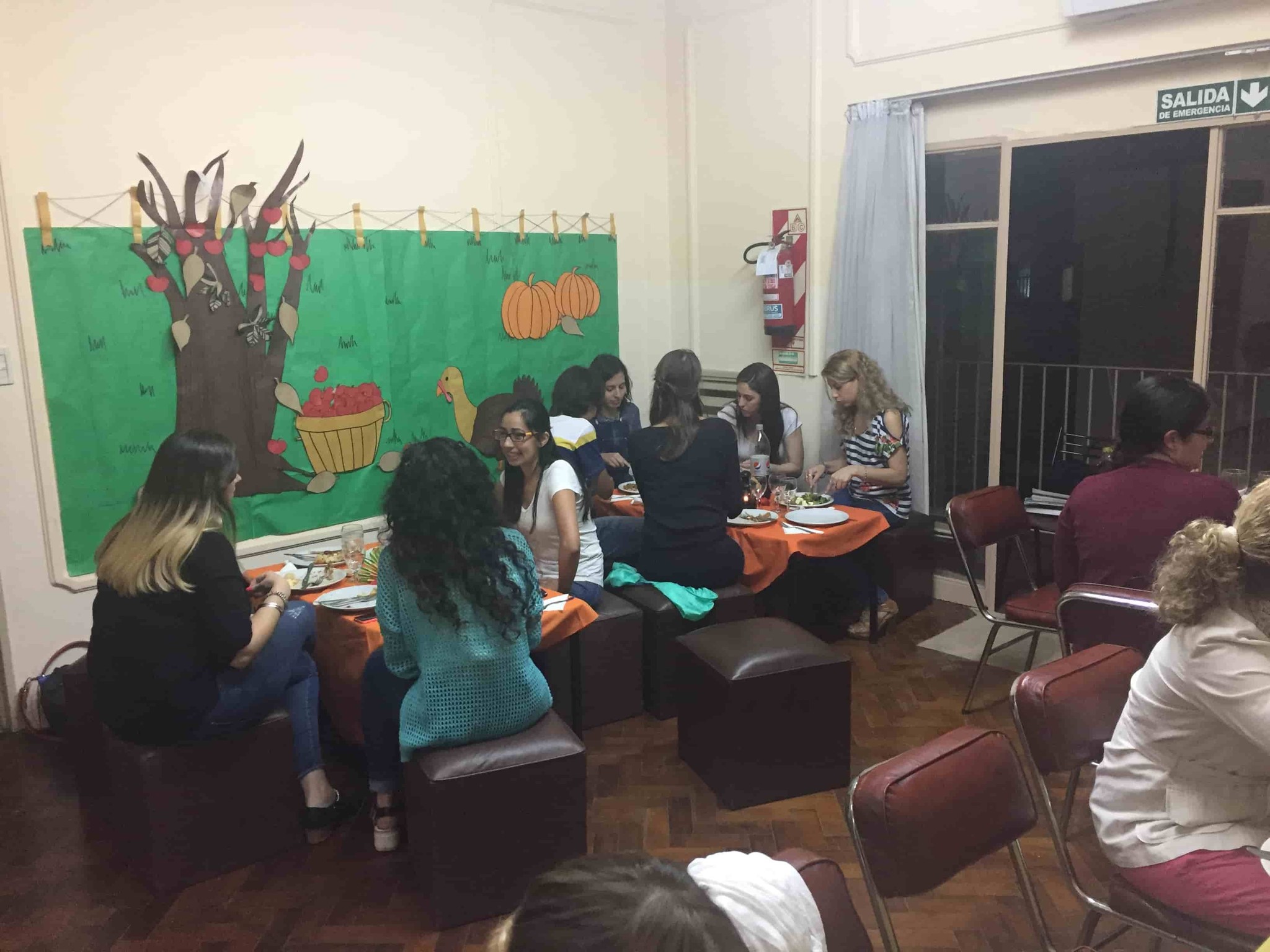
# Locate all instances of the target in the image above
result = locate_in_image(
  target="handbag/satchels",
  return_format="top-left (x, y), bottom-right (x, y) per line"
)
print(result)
top-left (37, 653), bottom-right (96, 739)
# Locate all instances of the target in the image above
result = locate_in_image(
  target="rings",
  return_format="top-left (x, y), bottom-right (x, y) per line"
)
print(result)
top-left (828, 479), bottom-right (832, 483)
top-left (805, 473), bottom-right (808, 479)
top-left (807, 474), bottom-right (813, 476)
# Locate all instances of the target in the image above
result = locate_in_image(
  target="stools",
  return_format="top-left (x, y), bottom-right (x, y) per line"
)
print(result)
top-left (529, 588), bottom-right (644, 729)
top-left (602, 583), bottom-right (756, 721)
top-left (75, 705), bottom-right (306, 896)
top-left (404, 706), bottom-right (588, 932)
top-left (810, 510), bottom-right (935, 627)
top-left (672, 616), bottom-right (853, 810)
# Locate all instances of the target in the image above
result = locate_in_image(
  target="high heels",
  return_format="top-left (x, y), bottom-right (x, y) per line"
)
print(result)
top-left (847, 596), bottom-right (900, 640)
top-left (302, 789), bottom-right (356, 842)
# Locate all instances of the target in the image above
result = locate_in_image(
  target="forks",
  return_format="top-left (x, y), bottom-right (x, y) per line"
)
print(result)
top-left (778, 521), bottom-right (823, 535)
top-left (813, 490), bottom-right (827, 500)
top-left (810, 484), bottom-right (815, 495)
top-left (300, 577), bottom-right (323, 590)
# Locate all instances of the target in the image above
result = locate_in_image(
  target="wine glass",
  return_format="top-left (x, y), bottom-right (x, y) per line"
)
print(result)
top-left (749, 472), bottom-right (797, 516)
top-left (341, 523), bottom-right (366, 585)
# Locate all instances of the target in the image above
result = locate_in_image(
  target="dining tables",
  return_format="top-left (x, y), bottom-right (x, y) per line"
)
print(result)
top-left (242, 540), bottom-right (600, 748)
top-left (590, 488), bottom-right (890, 645)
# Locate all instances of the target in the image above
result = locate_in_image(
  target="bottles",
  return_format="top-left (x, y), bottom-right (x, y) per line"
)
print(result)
top-left (749, 423), bottom-right (771, 500)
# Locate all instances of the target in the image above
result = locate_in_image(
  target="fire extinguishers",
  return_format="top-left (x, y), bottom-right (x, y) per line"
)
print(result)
top-left (762, 230), bottom-right (795, 336)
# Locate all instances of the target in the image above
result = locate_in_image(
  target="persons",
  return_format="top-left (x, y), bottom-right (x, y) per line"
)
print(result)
top-left (1051, 375), bottom-right (1243, 654)
top-left (492, 397), bottom-right (605, 677)
top-left (803, 347), bottom-right (914, 642)
top-left (361, 435), bottom-right (553, 852)
top-left (84, 427), bottom-right (367, 829)
top-left (486, 849), bottom-right (750, 952)
top-left (588, 353), bottom-right (642, 519)
top-left (546, 364), bottom-right (615, 520)
top-left (623, 348), bottom-right (746, 590)
top-left (716, 362), bottom-right (805, 500)
top-left (1086, 474), bottom-right (1270, 940)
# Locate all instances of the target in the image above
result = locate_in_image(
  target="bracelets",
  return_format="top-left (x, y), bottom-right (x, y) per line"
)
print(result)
top-left (257, 602), bottom-right (284, 616)
top-left (819, 463), bottom-right (828, 479)
top-left (863, 466), bottom-right (867, 480)
top-left (262, 591), bottom-right (287, 610)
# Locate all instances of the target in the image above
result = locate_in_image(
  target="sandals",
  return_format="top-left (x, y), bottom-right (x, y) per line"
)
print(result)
top-left (370, 802), bottom-right (402, 852)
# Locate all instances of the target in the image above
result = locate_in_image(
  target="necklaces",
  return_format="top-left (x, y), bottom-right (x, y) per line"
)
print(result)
top-left (600, 409), bottom-right (619, 422)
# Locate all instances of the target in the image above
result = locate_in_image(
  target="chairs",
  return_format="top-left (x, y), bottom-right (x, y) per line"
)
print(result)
top-left (1054, 582), bottom-right (1177, 660)
top-left (946, 485), bottom-right (1061, 715)
top-left (1010, 643), bottom-right (1270, 952)
top-left (773, 846), bottom-right (875, 952)
top-left (844, 724), bottom-right (1058, 952)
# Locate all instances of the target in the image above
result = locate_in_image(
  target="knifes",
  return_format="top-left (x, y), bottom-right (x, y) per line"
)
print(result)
top-left (332, 593), bottom-right (378, 607)
top-left (302, 563), bottom-right (315, 587)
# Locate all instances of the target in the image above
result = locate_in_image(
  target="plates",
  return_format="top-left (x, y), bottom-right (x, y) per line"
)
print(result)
top-left (785, 508), bottom-right (850, 525)
top-left (618, 480), bottom-right (640, 494)
top-left (290, 566), bottom-right (346, 592)
top-left (319, 585), bottom-right (378, 611)
top-left (292, 546), bottom-right (347, 567)
top-left (774, 491), bottom-right (834, 507)
top-left (726, 509), bottom-right (778, 527)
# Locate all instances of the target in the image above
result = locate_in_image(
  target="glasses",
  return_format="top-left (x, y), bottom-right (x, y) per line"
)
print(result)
top-left (1191, 426), bottom-right (1214, 443)
top-left (492, 428), bottom-right (541, 443)
